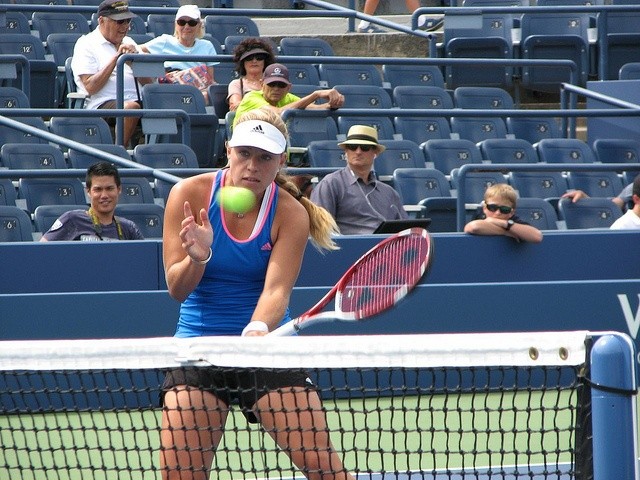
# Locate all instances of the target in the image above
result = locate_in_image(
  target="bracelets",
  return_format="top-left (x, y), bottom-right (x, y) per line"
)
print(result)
top-left (241, 321), bottom-right (268, 336)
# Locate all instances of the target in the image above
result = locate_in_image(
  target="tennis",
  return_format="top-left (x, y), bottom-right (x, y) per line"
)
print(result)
top-left (215, 187), bottom-right (254, 210)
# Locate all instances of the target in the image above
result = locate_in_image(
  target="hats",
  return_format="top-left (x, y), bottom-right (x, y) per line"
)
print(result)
top-left (337, 124), bottom-right (386, 154)
top-left (262, 62), bottom-right (290, 86)
top-left (227, 119), bottom-right (287, 155)
top-left (240, 46), bottom-right (271, 62)
top-left (175, 4), bottom-right (201, 21)
top-left (97, 0), bottom-right (138, 21)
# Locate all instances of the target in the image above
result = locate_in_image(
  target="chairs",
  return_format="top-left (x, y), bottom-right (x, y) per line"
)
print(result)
top-left (0, 115), bottom-right (48, 141)
top-left (617, 64), bottom-right (640, 79)
top-left (481, 138), bottom-right (539, 164)
top-left (453, 87), bottom-right (517, 115)
top-left (339, 117), bottom-right (393, 140)
top-left (384, 65), bottom-right (445, 87)
top-left (539, 138), bottom-right (594, 163)
top-left (1, 1), bottom-right (71, 8)
top-left (279, 38), bottom-right (335, 56)
top-left (423, 138), bottom-right (480, 176)
top-left (508, 171), bottom-right (568, 198)
top-left (463, 1), bottom-right (530, 11)
top-left (127, 33), bottom-right (154, 44)
top-left (0, 142), bottom-right (67, 177)
top-left (287, 84), bottom-right (327, 109)
top-left (141, 82), bottom-right (220, 165)
top-left (594, 9), bottom-right (640, 78)
top-left (210, 83), bottom-right (243, 164)
top-left (570, 168), bottom-right (621, 194)
top-left (96, 0), bottom-right (181, 9)
top-left (0, 35), bottom-right (59, 108)
top-left (286, 115), bottom-right (339, 148)
top-left (223, 34), bottom-right (282, 56)
top-left (201, 34), bottom-right (224, 55)
top-left (623, 171), bottom-right (639, 210)
top-left (394, 117), bottom-right (451, 139)
top-left (451, 168), bottom-right (508, 204)
top-left (51, 115), bottom-right (114, 144)
top-left (35, 204), bottom-right (90, 239)
top-left (32, 12), bottom-right (88, 41)
top-left (560, 196), bottom-right (623, 230)
top-left (392, 86), bottom-right (451, 111)
top-left (0, 87), bottom-right (30, 115)
top-left (1, 178), bottom-right (17, 206)
top-left (472, 198), bottom-right (558, 232)
top-left (392, 166), bottom-right (456, 231)
top-left (309, 139), bottom-right (349, 168)
top-left (204, 15), bottom-right (261, 46)
top-left (114, 177), bottom-right (155, 206)
top-left (0, 10), bottom-right (32, 34)
top-left (507, 117), bottom-right (562, 142)
top-left (317, 64), bottom-right (385, 87)
top-left (441, 9), bottom-right (513, 89)
top-left (20, 176), bottom-right (86, 213)
top-left (111, 204), bottom-right (166, 237)
top-left (91, 11), bottom-right (146, 34)
top-left (593, 136), bottom-right (638, 162)
top-left (134, 143), bottom-right (201, 202)
top-left (452, 117), bottom-right (507, 138)
top-left (47, 33), bottom-right (84, 66)
top-left (68, 143), bottom-right (136, 184)
top-left (279, 61), bottom-right (317, 84)
top-left (0, 205), bottom-right (33, 242)
top-left (372, 138), bottom-right (424, 175)
top-left (147, 13), bottom-right (175, 35)
top-left (538, 0), bottom-right (597, 8)
top-left (334, 85), bottom-right (390, 111)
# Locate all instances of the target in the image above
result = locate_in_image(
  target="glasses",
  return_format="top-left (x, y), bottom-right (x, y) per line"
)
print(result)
top-left (176, 19), bottom-right (200, 26)
top-left (485, 202), bottom-right (514, 214)
top-left (245, 53), bottom-right (265, 61)
top-left (345, 144), bottom-right (375, 152)
top-left (267, 82), bottom-right (287, 88)
top-left (111, 18), bottom-right (132, 25)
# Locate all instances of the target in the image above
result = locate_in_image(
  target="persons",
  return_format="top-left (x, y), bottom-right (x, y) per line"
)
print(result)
top-left (232, 63), bottom-right (345, 163)
top-left (137, 4), bottom-right (220, 106)
top-left (356, 0), bottom-right (444, 33)
top-left (40, 161), bottom-right (145, 241)
top-left (309, 125), bottom-right (410, 235)
top-left (157, 109), bottom-right (355, 480)
top-left (226, 37), bottom-right (277, 111)
top-left (560, 169), bottom-right (633, 209)
top-left (70, 0), bottom-right (147, 148)
top-left (609, 175), bottom-right (640, 231)
top-left (464, 183), bottom-right (543, 243)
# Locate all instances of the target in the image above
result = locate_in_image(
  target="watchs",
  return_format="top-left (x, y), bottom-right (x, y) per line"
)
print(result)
top-left (506, 219), bottom-right (514, 231)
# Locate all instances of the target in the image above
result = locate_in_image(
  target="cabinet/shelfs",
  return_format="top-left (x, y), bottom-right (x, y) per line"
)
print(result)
top-left (518, 12), bottom-right (588, 99)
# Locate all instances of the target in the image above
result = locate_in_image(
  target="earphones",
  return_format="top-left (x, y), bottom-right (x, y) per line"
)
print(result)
top-left (344, 153), bottom-right (347, 157)
top-left (373, 154), bottom-right (376, 159)
